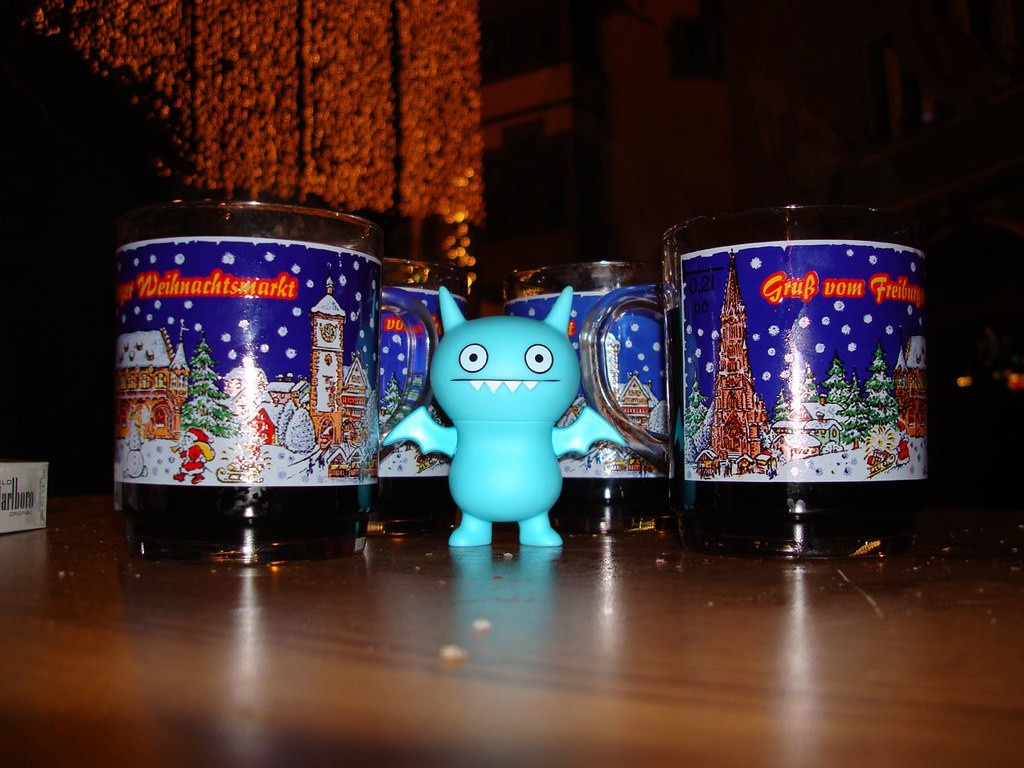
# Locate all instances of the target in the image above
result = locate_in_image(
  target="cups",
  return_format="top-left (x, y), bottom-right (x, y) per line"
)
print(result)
top-left (374, 258), bottom-right (479, 535)
top-left (580, 202), bottom-right (934, 556)
top-left (107, 203), bottom-right (383, 559)
top-left (500, 261), bottom-right (670, 533)
